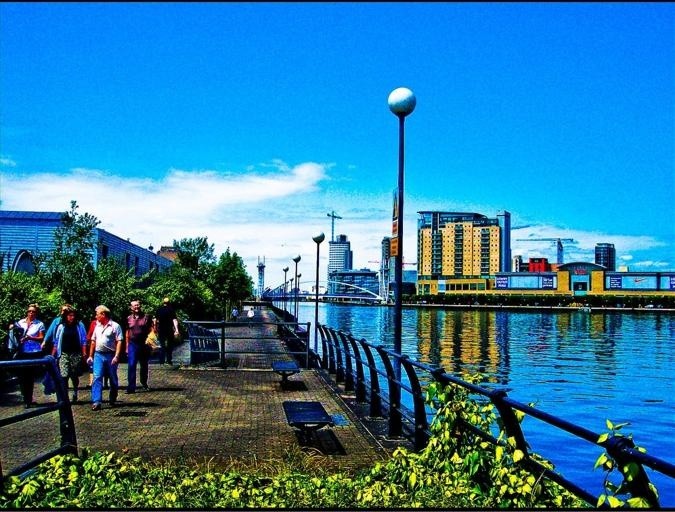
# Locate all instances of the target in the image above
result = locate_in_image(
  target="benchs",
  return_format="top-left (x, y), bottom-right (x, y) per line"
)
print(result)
top-left (283, 401), bottom-right (331, 445)
top-left (271, 360), bottom-right (300, 389)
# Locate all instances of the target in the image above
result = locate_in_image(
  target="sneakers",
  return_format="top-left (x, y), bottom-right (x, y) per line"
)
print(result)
top-left (142, 383), bottom-right (149, 392)
top-left (73, 394), bottom-right (77, 400)
top-left (92, 403), bottom-right (100, 410)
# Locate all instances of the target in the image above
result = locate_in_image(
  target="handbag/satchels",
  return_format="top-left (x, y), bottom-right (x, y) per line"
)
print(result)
top-left (8, 328), bottom-right (24, 351)
top-left (42, 373), bottom-right (55, 394)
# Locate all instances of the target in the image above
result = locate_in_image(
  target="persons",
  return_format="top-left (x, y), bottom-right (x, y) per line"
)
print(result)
top-left (41, 304), bottom-right (70, 356)
top-left (153, 298), bottom-right (180, 368)
top-left (123, 300), bottom-right (153, 394)
top-left (231, 307), bottom-right (240, 322)
top-left (86, 307), bottom-right (124, 411)
top-left (246, 307), bottom-right (255, 329)
top-left (54, 307), bottom-right (87, 404)
top-left (85, 304), bottom-right (109, 389)
top-left (8, 304), bottom-right (46, 409)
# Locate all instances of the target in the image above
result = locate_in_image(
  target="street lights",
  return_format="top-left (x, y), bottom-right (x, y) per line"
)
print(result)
top-left (312, 230), bottom-right (324, 352)
top-left (267, 254), bottom-right (301, 329)
top-left (388, 85), bottom-right (417, 407)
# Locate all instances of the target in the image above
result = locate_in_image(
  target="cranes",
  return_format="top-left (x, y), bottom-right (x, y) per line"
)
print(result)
top-left (326, 209), bottom-right (342, 242)
top-left (516, 237), bottom-right (574, 263)
top-left (367, 255), bottom-right (417, 270)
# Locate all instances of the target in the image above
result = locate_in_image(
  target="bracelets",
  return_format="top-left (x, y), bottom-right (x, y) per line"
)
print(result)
top-left (115, 356), bottom-right (119, 360)
